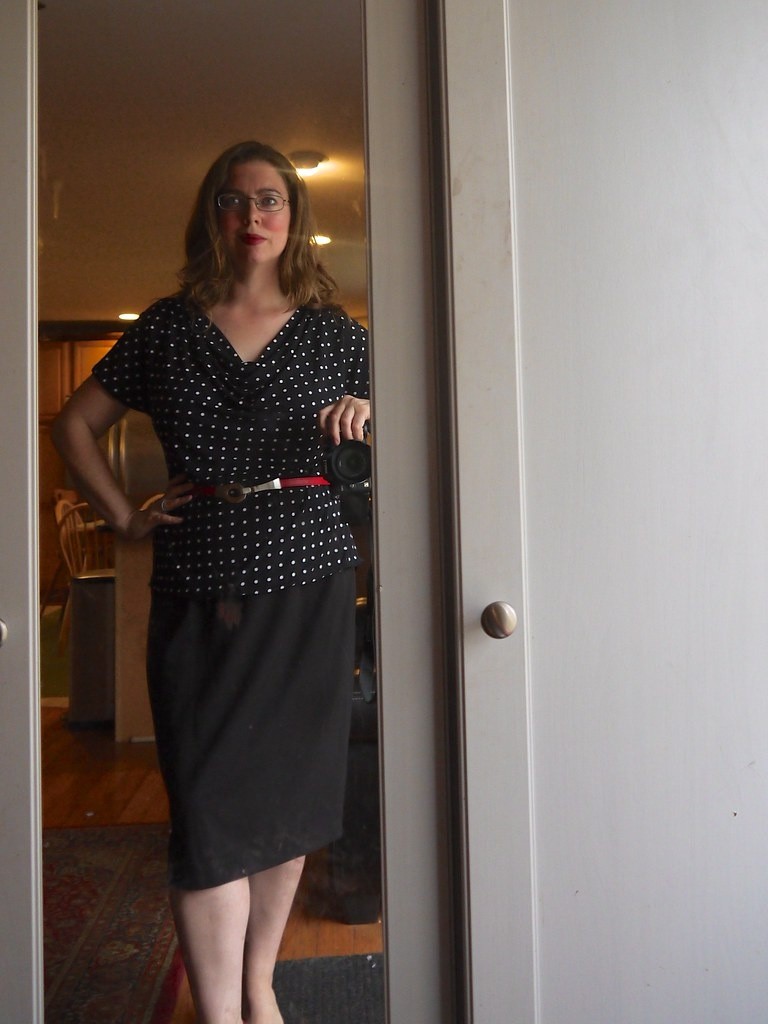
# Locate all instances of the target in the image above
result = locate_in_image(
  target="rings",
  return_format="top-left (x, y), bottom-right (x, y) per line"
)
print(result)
top-left (161, 499), bottom-right (166, 512)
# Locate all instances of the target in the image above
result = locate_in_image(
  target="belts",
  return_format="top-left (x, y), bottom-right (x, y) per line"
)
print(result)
top-left (188, 476), bottom-right (332, 502)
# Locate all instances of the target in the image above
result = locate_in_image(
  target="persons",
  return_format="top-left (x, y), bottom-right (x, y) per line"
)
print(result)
top-left (50, 140), bottom-right (375, 1024)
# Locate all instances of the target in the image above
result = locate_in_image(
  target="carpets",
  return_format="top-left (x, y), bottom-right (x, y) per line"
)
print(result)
top-left (271, 954), bottom-right (390, 1023)
top-left (40, 602), bottom-right (76, 697)
top-left (42, 823), bottom-right (187, 1024)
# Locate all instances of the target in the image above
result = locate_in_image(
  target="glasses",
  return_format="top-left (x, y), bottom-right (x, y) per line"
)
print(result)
top-left (218, 193), bottom-right (294, 212)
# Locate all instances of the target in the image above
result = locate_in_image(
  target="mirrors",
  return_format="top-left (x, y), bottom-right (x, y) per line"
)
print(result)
top-left (37, 1), bottom-right (390, 1024)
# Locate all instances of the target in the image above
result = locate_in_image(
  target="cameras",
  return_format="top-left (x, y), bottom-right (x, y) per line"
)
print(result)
top-left (321, 421), bottom-right (373, 496)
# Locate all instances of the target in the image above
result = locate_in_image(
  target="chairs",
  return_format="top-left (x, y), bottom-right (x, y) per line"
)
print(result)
top-left (53, 501), bottom-right (117, 726)
